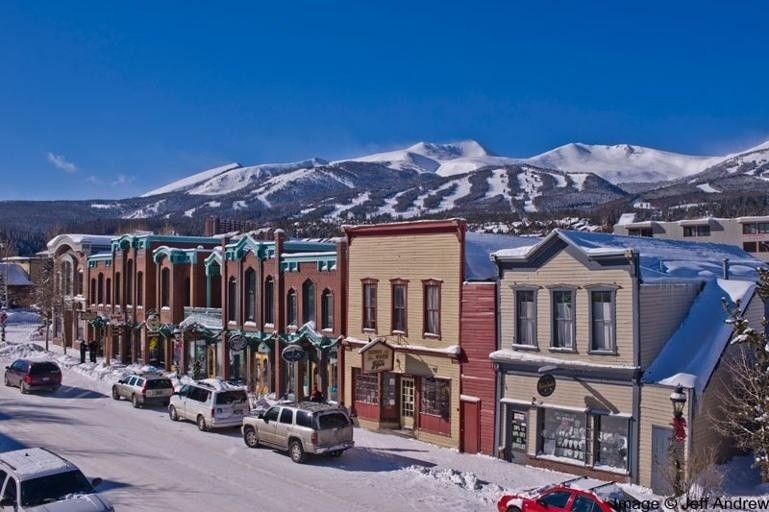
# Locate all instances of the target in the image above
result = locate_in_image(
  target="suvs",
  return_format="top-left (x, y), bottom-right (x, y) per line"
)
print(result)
top-left (110, 369), bottom-right (177, 410)
top-left (0, 444), bottom-right (114, 512)
top-left (238, 399), bottom-right (357, 465)
top-left (168, 378), bottom-right (253, 433)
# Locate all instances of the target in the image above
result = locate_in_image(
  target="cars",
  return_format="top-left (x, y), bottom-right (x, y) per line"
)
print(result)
top-left (496, 472), bottom-right (662, 512)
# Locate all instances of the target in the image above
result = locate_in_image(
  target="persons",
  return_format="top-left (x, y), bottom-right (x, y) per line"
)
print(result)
top-left (309, 390), bottom-right (323, 401)
top-left (89, 337), bottom-right (98, 362)
top-left (79, 340), bottom-right (88, 363)
top-left (336, 400), bottom-right (350, 415)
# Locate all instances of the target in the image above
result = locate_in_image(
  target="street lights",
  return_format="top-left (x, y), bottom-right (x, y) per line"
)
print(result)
top-left (670, 382), bottom-right (689, 491)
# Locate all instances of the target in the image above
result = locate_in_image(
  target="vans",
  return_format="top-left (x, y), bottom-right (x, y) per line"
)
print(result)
top-left (4, 357), bottom-right (63, 394)
